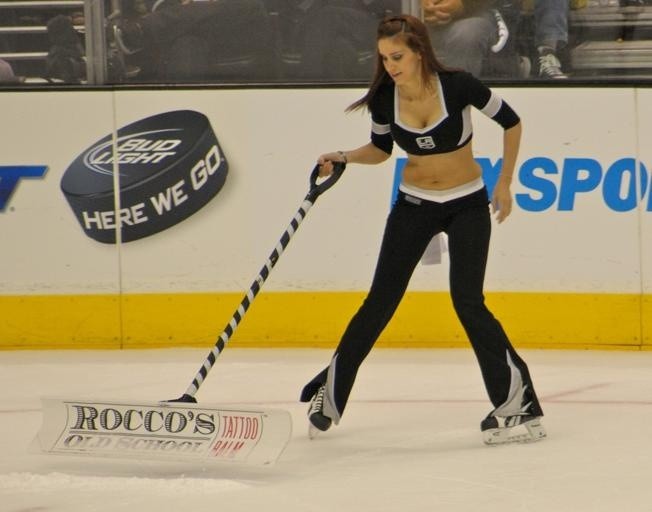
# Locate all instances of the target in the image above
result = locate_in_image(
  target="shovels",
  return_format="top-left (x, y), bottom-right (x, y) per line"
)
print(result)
top-left (49, 159), bottom-right (347, 472)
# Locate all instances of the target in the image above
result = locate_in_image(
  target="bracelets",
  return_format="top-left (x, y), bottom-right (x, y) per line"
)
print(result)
top-left (337, 150), bottom-right (348, 164)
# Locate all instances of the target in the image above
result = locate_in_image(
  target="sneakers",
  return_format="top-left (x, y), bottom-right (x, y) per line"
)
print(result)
top-left (516, 56), bottom-right (531, 77)
top-left (308, 384), bottom-right (331, 431)
top-left (114, 24), bottom-right (146, 55)
top-left (481, 414), bottom-right (540, 429)
top-left (536, 47), bottom-right (568, 78)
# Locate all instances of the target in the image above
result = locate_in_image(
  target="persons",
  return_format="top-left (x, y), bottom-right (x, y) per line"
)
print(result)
top-left (0, 1), bottom-right (572, 83)
top-left (296, 12), bottom-right (545, 433)
